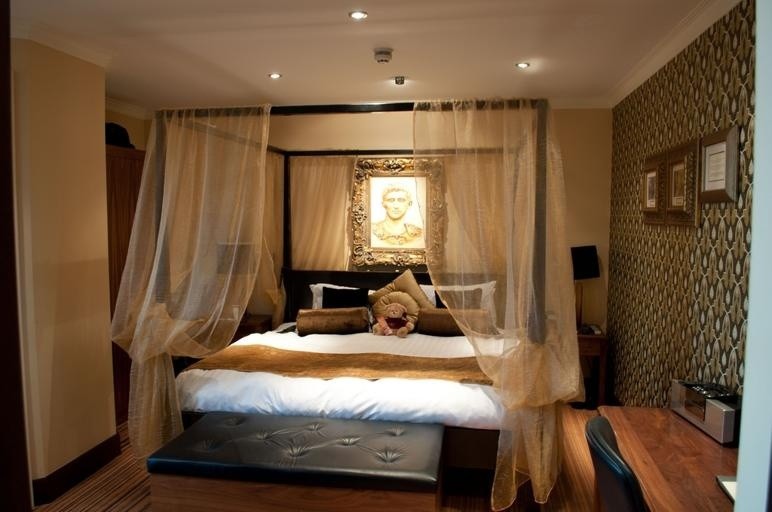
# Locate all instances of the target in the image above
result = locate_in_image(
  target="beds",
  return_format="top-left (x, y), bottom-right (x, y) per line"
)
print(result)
top-left (169, 268), bottom-right (521, 442)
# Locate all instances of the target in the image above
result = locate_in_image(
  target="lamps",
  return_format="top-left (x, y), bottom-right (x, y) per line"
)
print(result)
top-left (571, 244), bottom-right (601, 328)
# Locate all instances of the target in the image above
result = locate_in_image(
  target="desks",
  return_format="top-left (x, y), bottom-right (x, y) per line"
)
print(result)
top-left (596, 396), bottom-right (736, 512)
top-left (571, 327), bottom-right (606, 397)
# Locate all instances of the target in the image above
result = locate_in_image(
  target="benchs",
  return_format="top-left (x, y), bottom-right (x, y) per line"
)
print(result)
top-left (145, 409), bottom-right (446, 512)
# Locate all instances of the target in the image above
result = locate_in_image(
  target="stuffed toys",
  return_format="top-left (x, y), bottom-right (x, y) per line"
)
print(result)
top-left (376, 303), bottom-right (414, 337)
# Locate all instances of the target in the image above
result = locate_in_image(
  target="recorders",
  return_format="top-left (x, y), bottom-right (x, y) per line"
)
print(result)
top-left (670, 379), bottom-right (742, 445)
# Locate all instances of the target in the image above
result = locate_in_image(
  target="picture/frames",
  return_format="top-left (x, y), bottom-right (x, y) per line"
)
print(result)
top-left (350, 155), bottom-right (448, 272)
top-left (640, 124), bottom-right (741, 229)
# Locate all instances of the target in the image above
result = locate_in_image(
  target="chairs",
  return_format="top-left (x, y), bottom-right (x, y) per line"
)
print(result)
top-left (585, 416), bottom-right (651, 512)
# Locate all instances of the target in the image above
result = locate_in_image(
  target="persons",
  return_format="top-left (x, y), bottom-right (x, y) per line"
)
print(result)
top-left (371, 185), bottom-right (424, 245)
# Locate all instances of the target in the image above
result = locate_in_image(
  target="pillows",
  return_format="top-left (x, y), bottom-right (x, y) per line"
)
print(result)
top-left (295, 268), bottom-right (500, 343)
